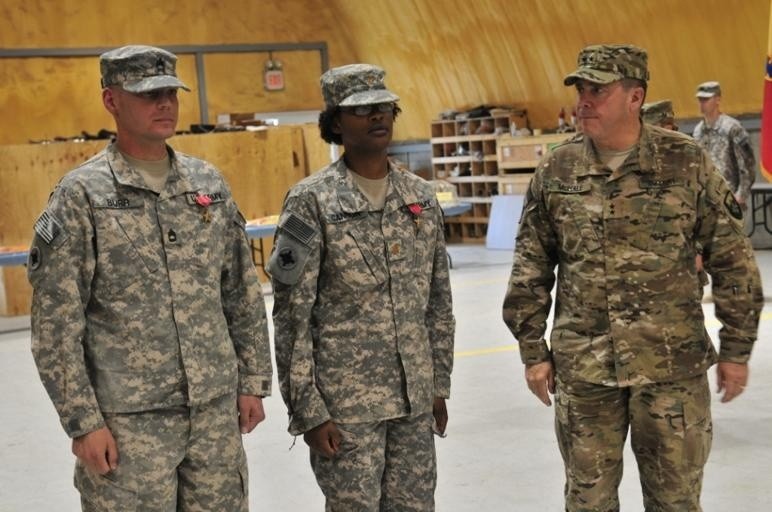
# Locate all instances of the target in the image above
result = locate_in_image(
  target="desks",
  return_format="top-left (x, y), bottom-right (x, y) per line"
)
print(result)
top-left (1, 250), bottom-right (31, 267)
top-left (439, 200), bottom-right (473, 270)
top-left (246, 215), bottom-right (283, 288)
top-left (746, 182), bottom-right (771, 238)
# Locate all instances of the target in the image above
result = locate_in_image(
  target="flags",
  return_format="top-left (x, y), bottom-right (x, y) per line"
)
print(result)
top-left (759, 1), bottom-right (772, 184)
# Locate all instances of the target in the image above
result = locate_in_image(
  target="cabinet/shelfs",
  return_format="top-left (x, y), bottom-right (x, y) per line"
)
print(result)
top-left (431, 111), bottom-right (527, 244)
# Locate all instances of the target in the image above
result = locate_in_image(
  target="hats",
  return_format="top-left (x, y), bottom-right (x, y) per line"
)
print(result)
top-left (318, 62), bottom-right (402, 112)
top-left (639, 98), bottom-right (676, 126)
top-left (695, 80), bottom-right (722, 99)
top-left (562, 42), bottom-right (651, 87)
top-left (98, 43), bottom-right (192, 95)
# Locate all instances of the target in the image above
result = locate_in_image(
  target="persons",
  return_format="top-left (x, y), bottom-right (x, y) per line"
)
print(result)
top-left (27, 43), bottom-right (277, 512)
top-left (686, 80), bottom-right (756, 238)
top-left (259, 61), bottom-right (457, 512)
top-left (498, 43), bottom-right (765, 512)
top-left (637, 99), bottom-right (678, 132)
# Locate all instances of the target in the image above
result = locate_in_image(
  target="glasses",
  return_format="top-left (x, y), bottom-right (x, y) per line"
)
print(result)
top-left (333, 102), bottom-right (397, 117)
